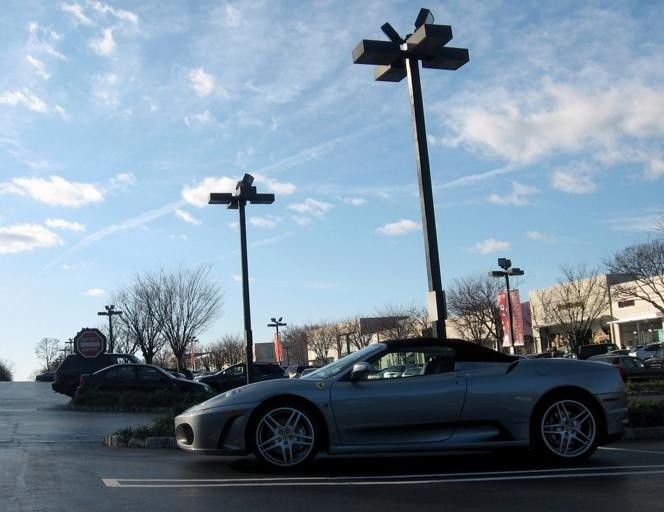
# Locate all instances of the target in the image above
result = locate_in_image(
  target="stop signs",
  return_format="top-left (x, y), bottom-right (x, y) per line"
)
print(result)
top-left (74, 328), bottom-right (107, 359)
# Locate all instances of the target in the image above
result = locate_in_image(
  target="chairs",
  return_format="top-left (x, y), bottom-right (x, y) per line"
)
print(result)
top-left (419, 357), bottom-right (434, 376)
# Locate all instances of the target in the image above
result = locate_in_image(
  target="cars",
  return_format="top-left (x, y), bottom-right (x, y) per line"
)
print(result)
top-left (73, 361), bottom-right (212, 403)
top-left (198, 360), bottom-right (290, 394)
top-left (34, 371), bottom-right (55, 382)
top-left (50, 352), bottom-right (187, 400)
top-left (515, 341), bottom-right (664, 382)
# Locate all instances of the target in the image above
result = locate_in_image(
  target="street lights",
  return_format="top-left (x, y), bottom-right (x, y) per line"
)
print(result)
top-left (56, 338), bottom-right (74, 364)
top-left (185, 334), bottom-right (200, 364)
top-left (96, 304), bottom-right (123, 353)
top-left (266, 316), bottom-right (288, 367)
top-left (205, 172), bottom-right (276, 363)
top-left (487, 255), bottom-right (524, 354)
top-left (353, 7), bottom-right (473, 338)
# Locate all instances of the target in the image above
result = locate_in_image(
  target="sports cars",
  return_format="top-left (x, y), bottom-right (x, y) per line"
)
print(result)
top-left (170, 336), bottom-right (631, 471)
